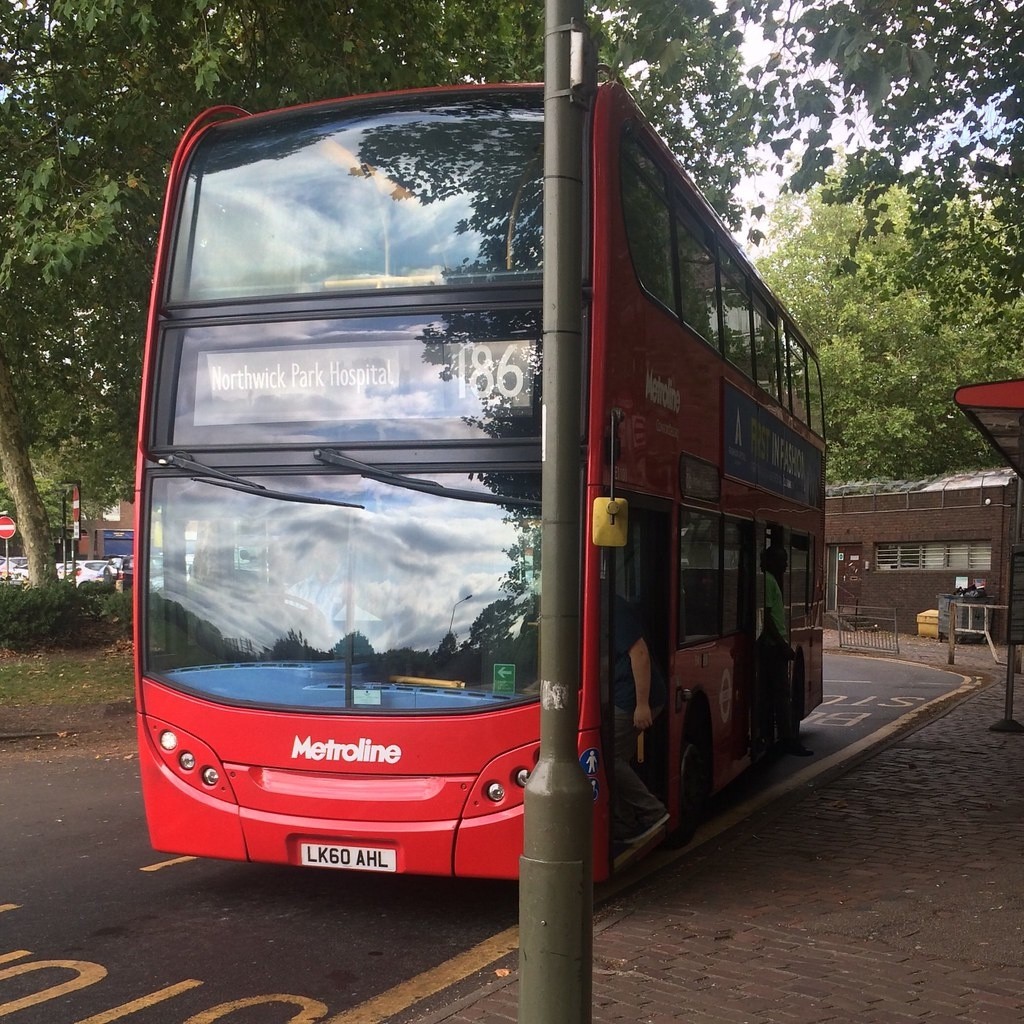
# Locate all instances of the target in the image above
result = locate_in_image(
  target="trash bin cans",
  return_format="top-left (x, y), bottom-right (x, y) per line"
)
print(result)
top-left (917, 608), bottom-right (938, 639)
top-left (937, 592), bottom-right (996, 644)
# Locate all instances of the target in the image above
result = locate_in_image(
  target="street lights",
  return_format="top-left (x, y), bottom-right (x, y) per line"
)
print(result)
top-left (448, 593), bottom-right (474, 634)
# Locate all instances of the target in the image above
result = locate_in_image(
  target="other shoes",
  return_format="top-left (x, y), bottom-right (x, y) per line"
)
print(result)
top-left (623, 810), bottom-right (671, 844)
top-left (787, 741), bottom-right (814, 757)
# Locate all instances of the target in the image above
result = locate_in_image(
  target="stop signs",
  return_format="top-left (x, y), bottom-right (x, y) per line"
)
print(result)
top-left (0, 515), bottom-right (17, 539)
top-left (72, 486), bottom-right (80, 522)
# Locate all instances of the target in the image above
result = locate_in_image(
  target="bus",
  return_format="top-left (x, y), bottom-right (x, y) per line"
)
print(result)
top-left (129, 60), bottom-right (829, 885)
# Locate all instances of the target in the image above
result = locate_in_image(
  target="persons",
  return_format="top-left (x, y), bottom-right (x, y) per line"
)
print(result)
top-left (286, 540), bottom-right (377, 622)
top-left (524, 593), bottom-right (670, 842)
top-left (763, 544), bottom-right (815, 758)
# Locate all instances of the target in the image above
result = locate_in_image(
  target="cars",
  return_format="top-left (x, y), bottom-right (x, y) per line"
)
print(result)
top-left (0, 553), bottom-right (197, 594)
